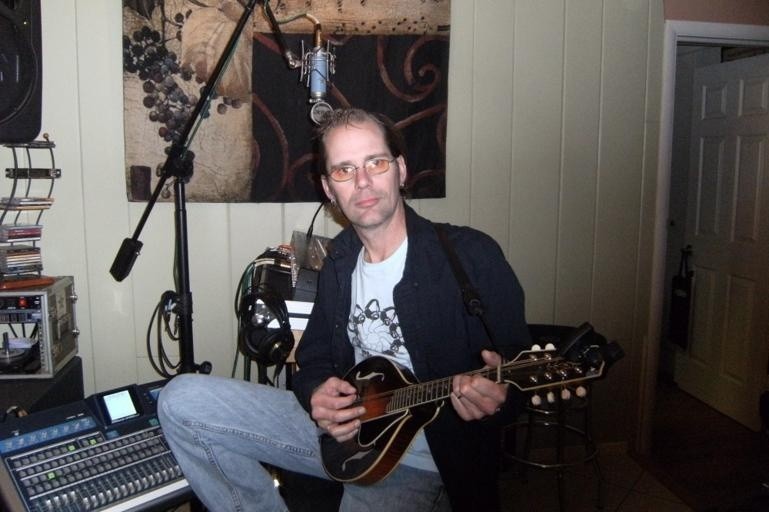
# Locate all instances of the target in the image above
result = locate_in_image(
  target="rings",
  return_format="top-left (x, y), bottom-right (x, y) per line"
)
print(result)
top-left (456, 394), bottom-right (464, 399)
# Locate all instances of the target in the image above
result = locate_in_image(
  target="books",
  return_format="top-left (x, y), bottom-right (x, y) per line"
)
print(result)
top-left (0, 138), bottom-right (61, 290)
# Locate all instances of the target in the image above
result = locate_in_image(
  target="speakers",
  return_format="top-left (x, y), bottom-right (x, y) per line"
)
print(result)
top-left (0, 0), bottom-right (43, 144)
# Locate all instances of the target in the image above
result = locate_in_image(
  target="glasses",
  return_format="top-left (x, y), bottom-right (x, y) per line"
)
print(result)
top-left (326, 157), bottom-right (396, 182)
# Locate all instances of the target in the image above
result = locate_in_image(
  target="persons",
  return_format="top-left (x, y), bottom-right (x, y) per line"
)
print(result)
top-left (154, 104), bottom-right (531, 511)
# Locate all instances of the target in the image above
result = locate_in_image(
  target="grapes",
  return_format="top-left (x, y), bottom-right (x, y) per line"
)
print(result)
top-left (123, 13), bottom-right (242, 198)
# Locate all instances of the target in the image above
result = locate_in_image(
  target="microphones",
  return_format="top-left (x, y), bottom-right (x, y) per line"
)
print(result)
top-left (308, 45), bottom-right (338, 129)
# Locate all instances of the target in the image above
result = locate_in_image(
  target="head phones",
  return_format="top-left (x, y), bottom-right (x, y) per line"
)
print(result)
top-left (236, 289), bottom-right (296, 368)
